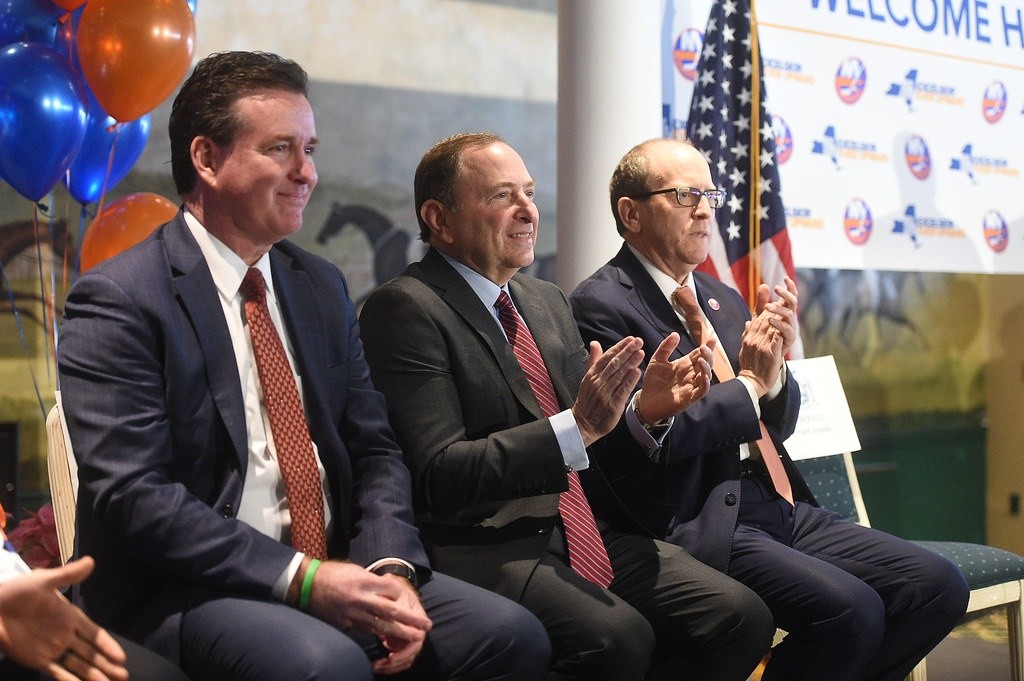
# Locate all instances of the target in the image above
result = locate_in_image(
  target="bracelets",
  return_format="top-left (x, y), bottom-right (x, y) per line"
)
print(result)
top-left (374, 564), bottom-right (419, 588)
top-left (298, 559), bottom-right (320, 610)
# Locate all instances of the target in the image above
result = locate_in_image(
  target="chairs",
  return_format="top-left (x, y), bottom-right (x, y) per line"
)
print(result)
top-left (782, 354), bottom-right (1024, 681)
top-left (44, 402), bottom-right (78, 565)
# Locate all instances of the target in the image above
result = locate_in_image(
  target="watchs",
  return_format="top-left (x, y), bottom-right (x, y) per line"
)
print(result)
top-left (634, 394), bottom-right (672, 437)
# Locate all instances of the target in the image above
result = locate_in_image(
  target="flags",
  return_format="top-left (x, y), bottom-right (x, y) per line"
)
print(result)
top-left (684, 0), bottom-right (801, 369)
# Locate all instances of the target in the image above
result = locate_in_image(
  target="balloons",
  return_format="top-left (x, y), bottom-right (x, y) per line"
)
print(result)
top-left (0, 0), bottom-right (199, 275)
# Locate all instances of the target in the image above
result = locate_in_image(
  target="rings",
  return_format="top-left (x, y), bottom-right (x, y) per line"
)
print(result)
top-left (58, 650), bottom-right (69, 664)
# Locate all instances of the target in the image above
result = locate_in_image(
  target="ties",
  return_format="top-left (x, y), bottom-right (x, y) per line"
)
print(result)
top-left (238, 268), bottom-right (329, 564)
top-left (492, 291), bottom-right (616, 588)
top-left (673, 285), bottom-right (796, 509)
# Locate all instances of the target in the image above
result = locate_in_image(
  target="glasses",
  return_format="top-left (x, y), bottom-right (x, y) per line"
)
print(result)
top-left (631, 187), bottom-right (727, 209)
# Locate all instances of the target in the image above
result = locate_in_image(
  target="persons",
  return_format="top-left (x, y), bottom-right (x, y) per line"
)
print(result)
top-left (358, 135), bottom-right (777, 681)
top-left (0, 533), bottom-right (186, 681)
top-left (57, 47), bottom-right (559, 681)
top-left (568, 137), bottom-right (971, 681)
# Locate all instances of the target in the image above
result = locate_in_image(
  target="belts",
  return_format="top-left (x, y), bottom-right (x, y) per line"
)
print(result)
top-left (738, 454), bottom-right (766, 478)
